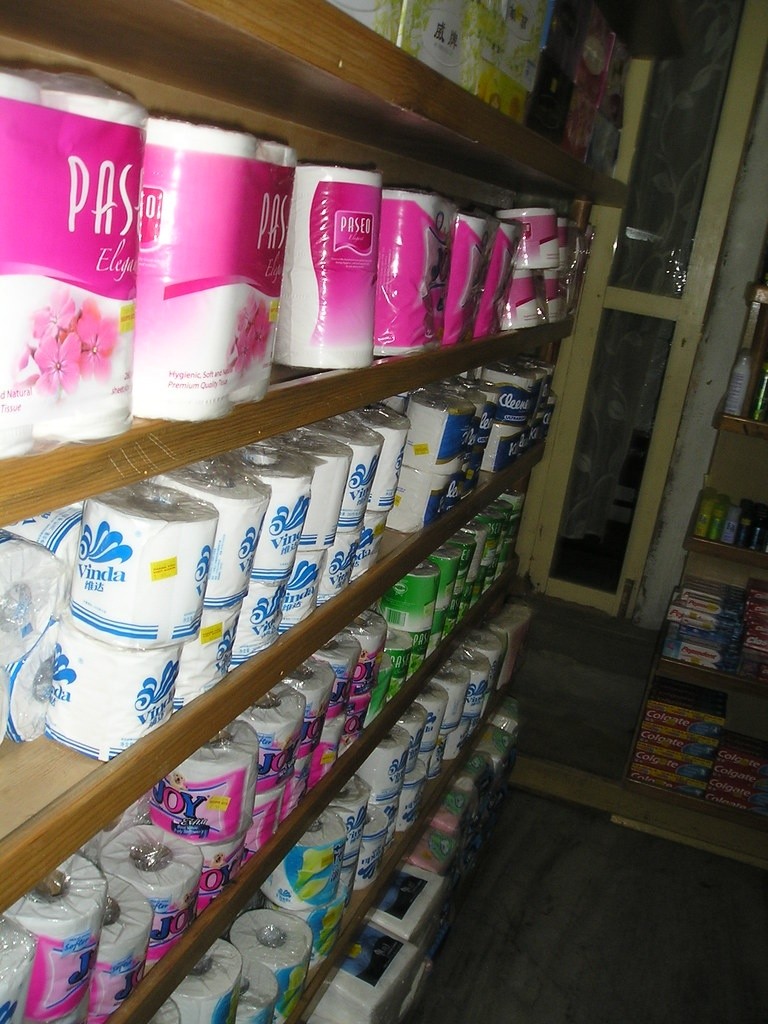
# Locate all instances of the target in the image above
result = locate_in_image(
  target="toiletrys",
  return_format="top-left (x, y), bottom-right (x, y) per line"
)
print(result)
top-left (619, 337), bottom-right (768, 813)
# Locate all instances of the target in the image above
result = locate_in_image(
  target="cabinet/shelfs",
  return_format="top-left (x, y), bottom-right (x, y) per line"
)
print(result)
top-left (624, 226), bottom-right (768, 828)
top-left (0, 0), bottom-right (630, 1024)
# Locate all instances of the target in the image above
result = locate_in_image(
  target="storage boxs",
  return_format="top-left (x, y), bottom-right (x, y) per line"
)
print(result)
top-left (629, 575), bottom-right (768, 815)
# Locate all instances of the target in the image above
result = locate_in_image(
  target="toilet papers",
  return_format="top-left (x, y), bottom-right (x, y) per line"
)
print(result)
top-left (0, 0), bottom-right (570, 1024)
